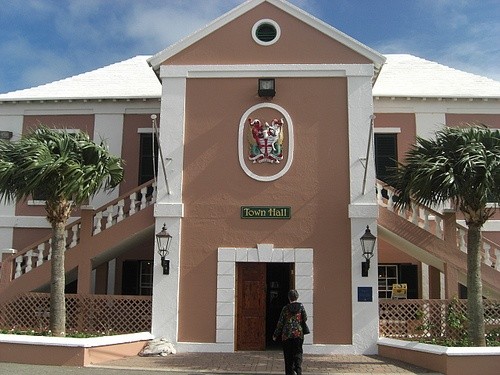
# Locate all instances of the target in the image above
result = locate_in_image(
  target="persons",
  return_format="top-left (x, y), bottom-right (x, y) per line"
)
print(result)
top-left (272, 289), bottom-right (311, 375)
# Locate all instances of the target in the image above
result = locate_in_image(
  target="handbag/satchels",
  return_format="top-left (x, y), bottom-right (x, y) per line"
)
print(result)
top-left (302, 319), bottom-right (310, 335)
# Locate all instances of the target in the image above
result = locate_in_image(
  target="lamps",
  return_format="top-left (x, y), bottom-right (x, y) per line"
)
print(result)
top-left (360, 225), bottom-right (376, 277)
top-left (156, 223), bottom-right (172, 275)
top-left (258, 78), bottom-right (276, 97)
